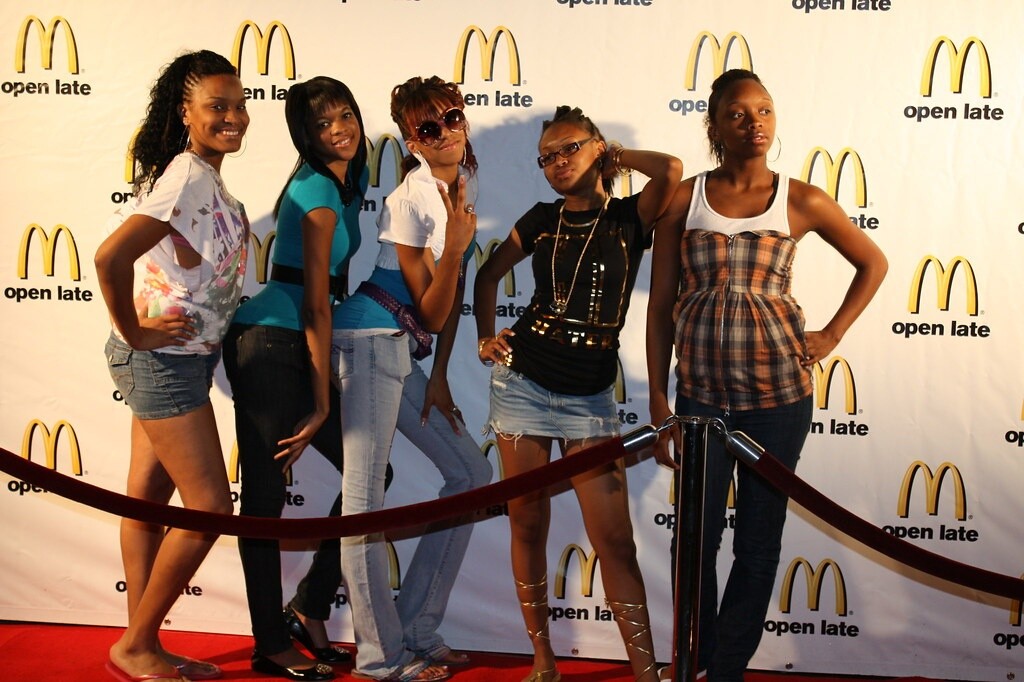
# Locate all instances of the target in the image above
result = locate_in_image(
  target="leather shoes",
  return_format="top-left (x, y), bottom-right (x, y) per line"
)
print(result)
top-left (282, 603), bottom-right (352, 662)
top-left (250, 651), bottom-right (333, 682)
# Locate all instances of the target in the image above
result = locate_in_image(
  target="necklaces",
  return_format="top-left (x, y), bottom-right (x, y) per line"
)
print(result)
top-left (549, 197), bottom-right (610, 315)
top-left (189, 148), bottom-right (220, 175)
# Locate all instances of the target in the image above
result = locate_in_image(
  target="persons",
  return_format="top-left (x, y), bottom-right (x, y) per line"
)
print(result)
top-left (329, 75), bottom-right (493, 682)
top-left (222, 76), bottom-right (371, 682)
top-left (646, 69), bottom-right (888, 682)
top-left (94, 49), bottom-right (250, 682)
top-left (474, 105), bottom-right (683, 682)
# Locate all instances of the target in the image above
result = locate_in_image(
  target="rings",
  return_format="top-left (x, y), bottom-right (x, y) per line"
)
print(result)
top-left (496, 335), bottom-right (500, 340)
top-left (452, 407), bottom-right (457, 412)
top-left (468, 207), bottom-right (472, 212)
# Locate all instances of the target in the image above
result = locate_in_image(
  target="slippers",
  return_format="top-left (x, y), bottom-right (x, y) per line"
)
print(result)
top-left (105, 660), bottom-right (185, 682)
top-left (351, 669), bottom-right (448, 681)
top-left (175, 656), bottom-right (220, 678)
top-left (430, 652), bottom-right (472, 664)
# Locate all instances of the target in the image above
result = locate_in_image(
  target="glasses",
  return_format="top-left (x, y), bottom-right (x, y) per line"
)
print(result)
top-left (538, 136), bottom-right (599, 169)
top-left (410, 106), bottom-right (465, 146)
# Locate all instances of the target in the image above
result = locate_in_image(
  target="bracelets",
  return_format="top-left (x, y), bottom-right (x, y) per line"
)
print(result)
top-left (612, 147), bottom-right (635, 176)
top-left (478, 337), bottom-right (496, 367)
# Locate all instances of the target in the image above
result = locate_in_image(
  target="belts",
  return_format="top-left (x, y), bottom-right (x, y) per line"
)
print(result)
top-left (523, 303), bottom-right (620, 349)
top-left (355, 279), bottom-right (433, 361)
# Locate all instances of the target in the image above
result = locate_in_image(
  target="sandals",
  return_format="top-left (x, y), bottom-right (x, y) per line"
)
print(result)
top-left (522, 664), bottom-right (560, 682)
top-left (656, 667), bottom-right (707, 682)
